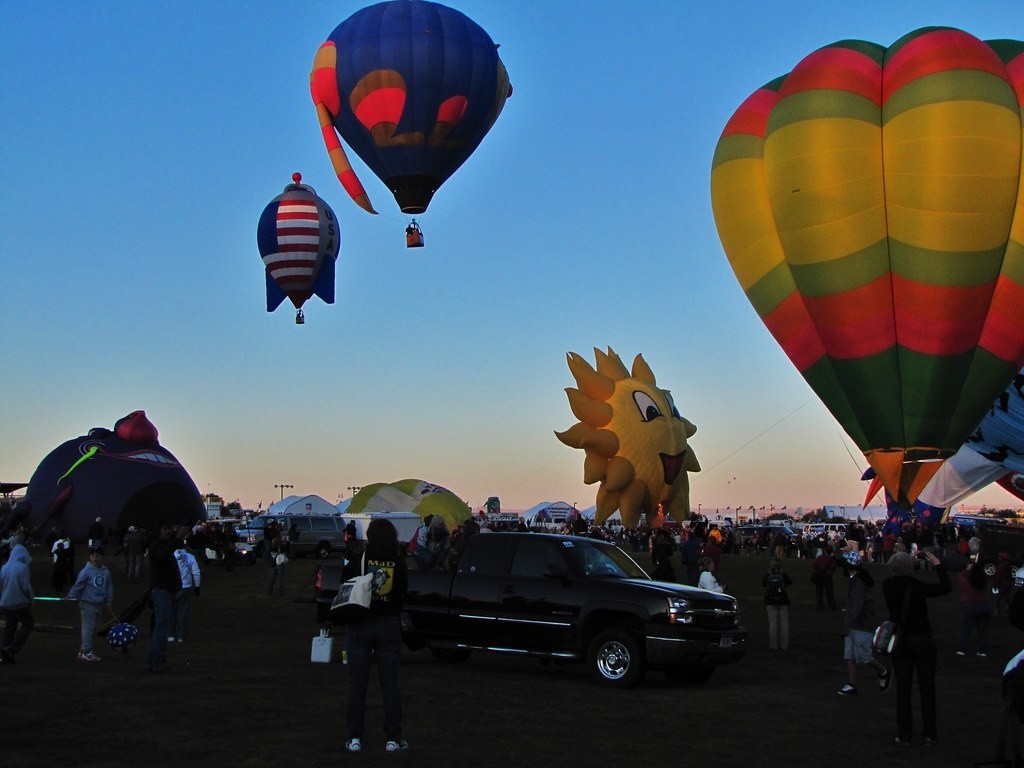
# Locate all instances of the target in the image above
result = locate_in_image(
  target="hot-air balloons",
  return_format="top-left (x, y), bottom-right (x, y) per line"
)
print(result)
top-left (710, 26), bottom-right (1024, 524)
top-left (309, 1), bottom-right (513, 247)
top-left (255, 173), bottom-right (342, 324)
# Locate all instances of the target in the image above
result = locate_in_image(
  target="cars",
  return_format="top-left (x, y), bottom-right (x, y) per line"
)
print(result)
top-left (738, 514), bottom-right (1024, 588)
top-left (400, 531), bottom-right (752, 690)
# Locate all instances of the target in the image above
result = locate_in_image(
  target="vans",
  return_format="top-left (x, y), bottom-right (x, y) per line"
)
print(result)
top-left (235, 515), bottom-right (349, 559)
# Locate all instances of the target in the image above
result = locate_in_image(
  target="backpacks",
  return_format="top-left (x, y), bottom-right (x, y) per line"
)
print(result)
top-left (766, 566), bottom-right (786, 601)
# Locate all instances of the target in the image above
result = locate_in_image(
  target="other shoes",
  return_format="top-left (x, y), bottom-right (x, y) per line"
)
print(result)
top-left (0, 650), bottom-right (18, 664)
top-left (923, 735), bottom-right (935, 746)
top-left (976, 648), bottom-right (993, 657)
top-left (887, 736), bottom-right (911, 746)
top-left (956, 648), bottom-right (968, 657)
top-left (167, 636), bottom-right (175, 642)
top-left (178, 637), bottom-right (187, 643)
top-left (143, 656), bottom-right (154, 671)
top-left (77, 650), bottom-right (102, 661)
top-left (153, 659), bottom-right (174, 674)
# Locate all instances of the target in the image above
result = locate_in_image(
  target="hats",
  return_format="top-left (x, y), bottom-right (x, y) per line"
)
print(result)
top-left (885, 551), bottom-right (913, 576)
top-left (998, 551), bottom-right (1010, 559)
top-left (96, 517), bottom-right (102, 523)
top-left (89, 545), bottom-right (106, 556)
top-left (128, 526), bottom-right (135, 532)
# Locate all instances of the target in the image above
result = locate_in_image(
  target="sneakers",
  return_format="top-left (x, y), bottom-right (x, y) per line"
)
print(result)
top-left (345, 737), bottom-right (362, 750)
top-left (879, 666), bottom-right (892, 692)
top-left (837, 682), bottom-right (859, 696)
top-left (385, 739), bottom-right (408, 751)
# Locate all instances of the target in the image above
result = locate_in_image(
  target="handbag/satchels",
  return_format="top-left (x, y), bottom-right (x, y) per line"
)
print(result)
top-left (275, 547), bottom-right (288, 566)
top-left (331, 553), bottom-right (374, 621)
top-left (810, 569), bottom-right (826, 584)
top-left (872, 620), bottom-right (899, 655)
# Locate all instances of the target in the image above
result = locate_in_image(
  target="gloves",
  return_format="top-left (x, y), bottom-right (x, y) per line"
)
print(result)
top-left (193, 586), bottom-right (200, 597)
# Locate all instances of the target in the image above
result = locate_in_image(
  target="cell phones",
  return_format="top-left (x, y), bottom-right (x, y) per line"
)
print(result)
top-left (917, 552), bottom-right (927, 559)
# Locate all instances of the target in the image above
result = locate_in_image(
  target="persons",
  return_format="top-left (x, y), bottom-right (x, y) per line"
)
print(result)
top-left (993, 586), bottom-right (1024, 768)
top-left (0, 544), bottom-right (36, 665)
top-left (0, 507), bottom-right (1024, 655)
top-left (341, 520), bottom-right (408, 753)
top-left (65, 545), bottom-right (113, 662)
top-left (882, 550), bottom-right (953, 746)
top-left (835, 550), bottom-right (893, 698)
top-left (140, 520), bottom-right (183, 674)
